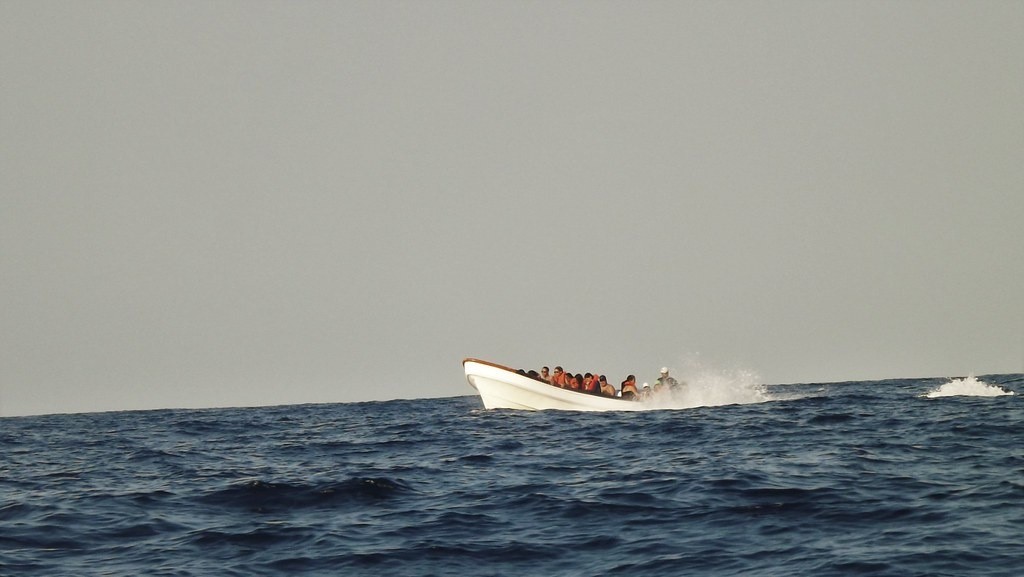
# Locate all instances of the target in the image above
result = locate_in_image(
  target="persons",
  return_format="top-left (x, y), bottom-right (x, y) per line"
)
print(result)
top-left (622, 375), bottom-right (641, 401)
top-left (643, 383), bottom-right (651, 394)
top-left (541, 367), bottom-right (554, 385)
top-left (553, 366), bottom-right (601, 394)
top-left (600, 375), bottom-right (615, 396)
top-left (654, 382), bottom-right (663, 393)
top-left (658, 368), bottom-right (677, 388)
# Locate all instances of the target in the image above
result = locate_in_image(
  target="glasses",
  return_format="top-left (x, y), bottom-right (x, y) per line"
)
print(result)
top-left (554, 371), bottom-right (560, 373)
top-left (541, 371), bottom-right (547, 373)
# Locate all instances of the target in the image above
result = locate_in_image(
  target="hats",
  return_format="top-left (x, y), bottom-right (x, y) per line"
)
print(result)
top-left (655, 380), bottom-right (661, 385)
top-left (643, 383), bottom-right (650, 388)
top-left (660, 367), bottom-right (668, 373)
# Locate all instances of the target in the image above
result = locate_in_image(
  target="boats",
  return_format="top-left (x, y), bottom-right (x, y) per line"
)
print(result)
top-left (462, 359), bottom-right (642, 412)
